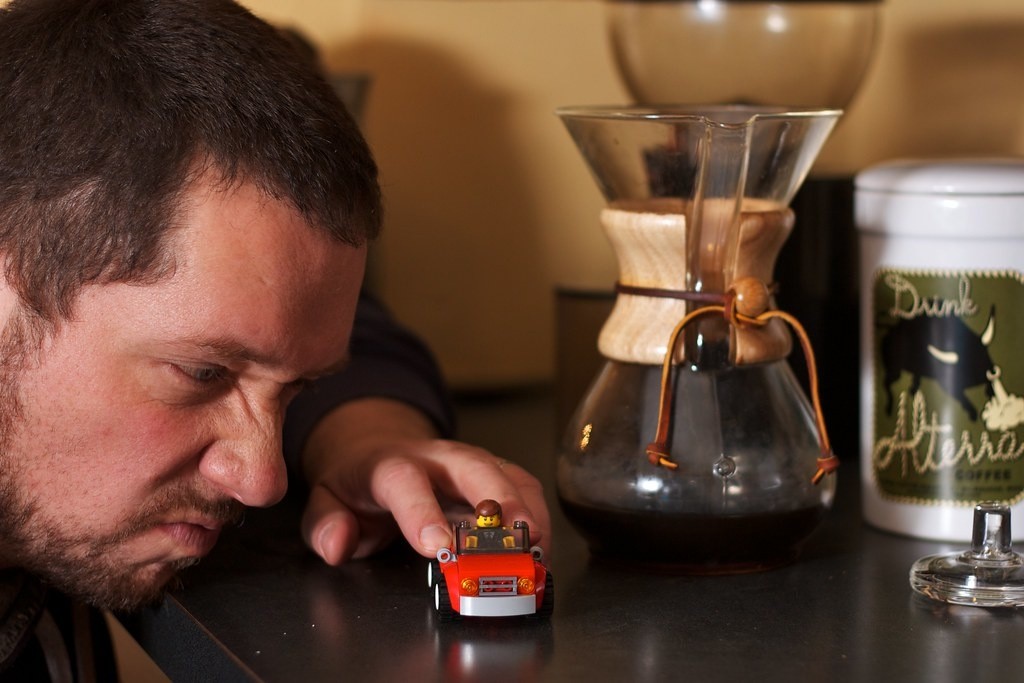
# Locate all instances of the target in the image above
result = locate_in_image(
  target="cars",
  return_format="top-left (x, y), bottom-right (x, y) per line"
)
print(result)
top-left (427, 519), bottom-right (554, 623)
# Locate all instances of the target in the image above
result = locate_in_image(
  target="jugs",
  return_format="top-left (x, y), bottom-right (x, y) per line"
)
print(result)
top-left (605, 1), bottom-right (883, 461)
top-left (555, 106), bottom-right (844, 579)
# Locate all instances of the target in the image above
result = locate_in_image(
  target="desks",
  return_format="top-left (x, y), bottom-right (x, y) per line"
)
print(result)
top-left (104, 390), bottom-right (1024, 683)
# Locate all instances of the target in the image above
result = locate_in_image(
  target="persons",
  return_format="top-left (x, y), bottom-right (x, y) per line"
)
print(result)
top-left (466, 499), bottom-right (515, 548)
top-left (0, 1), bottom-right (551, 683)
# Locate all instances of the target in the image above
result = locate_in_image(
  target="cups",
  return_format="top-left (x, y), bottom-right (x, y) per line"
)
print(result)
top-left (852, 160), bottom-right (1023, 544)
